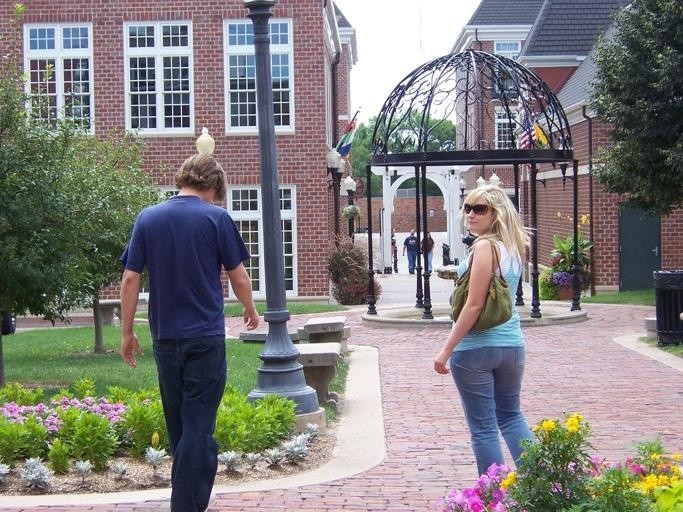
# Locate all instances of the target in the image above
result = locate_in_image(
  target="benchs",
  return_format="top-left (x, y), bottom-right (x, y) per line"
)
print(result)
top-left (292, 315), bottom-right (351, 410)
top-left (82, 298), bottom-right (121, 328)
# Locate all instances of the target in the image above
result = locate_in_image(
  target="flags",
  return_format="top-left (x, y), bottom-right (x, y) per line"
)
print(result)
top-left (520, 111), bottom-right (532, 174)
top-left (334, 112), bottom-right (356, 158)
top-left (529, 112), bottom-right (548, 150)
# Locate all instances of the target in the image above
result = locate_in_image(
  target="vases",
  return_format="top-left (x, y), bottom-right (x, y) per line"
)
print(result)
top-left (559, 284), bottom-right (574, 299)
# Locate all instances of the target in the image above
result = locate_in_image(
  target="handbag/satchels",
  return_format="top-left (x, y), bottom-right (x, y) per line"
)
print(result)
top-left (449, 272), bottom-right (513, 329)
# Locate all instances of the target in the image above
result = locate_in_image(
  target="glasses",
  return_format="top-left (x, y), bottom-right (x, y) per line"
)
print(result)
top-left (463, 203), bottom-right (492, 217)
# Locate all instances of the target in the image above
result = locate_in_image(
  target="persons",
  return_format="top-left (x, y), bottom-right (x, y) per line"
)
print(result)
top-left (402, 228), bottom-right (417, 274)
top-left (434, 185), bottom-right (542, 485)
top-left (116, 153), bottom-right (258, 511)
top-left (391, 228), bottom-right (398, 264)
top-left (420, 231), bottom-right (434, 273)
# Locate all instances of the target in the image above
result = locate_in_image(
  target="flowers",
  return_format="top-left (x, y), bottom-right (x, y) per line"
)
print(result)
top-left (544, 210), bottom-right (593, 287)
top-left (332, 279), bottom-right (369, 304)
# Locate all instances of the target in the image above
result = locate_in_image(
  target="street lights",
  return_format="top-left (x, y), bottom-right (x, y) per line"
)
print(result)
top-left (344, 176), bottom-right (357, 243)
top-left (326, 148), bottom-right (346, 246)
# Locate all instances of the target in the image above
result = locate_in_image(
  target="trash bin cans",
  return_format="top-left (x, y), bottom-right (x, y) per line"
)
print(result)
top-left (652, 269), bottom-right (683, 347)
top-left (0, 309), bottom-right (16, 336)
